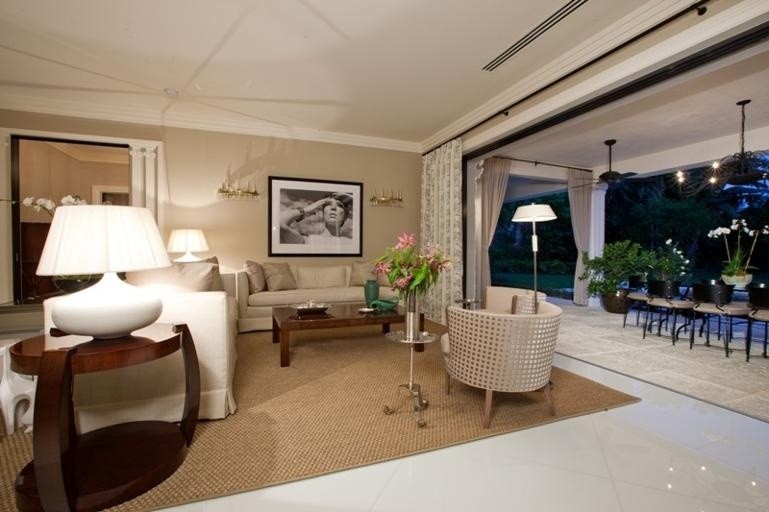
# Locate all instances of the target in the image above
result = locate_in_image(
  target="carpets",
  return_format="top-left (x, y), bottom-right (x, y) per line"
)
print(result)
top-left (555, 304), bottom-right (769, 425)
top-left (1, 319), bottom-right (643, 511)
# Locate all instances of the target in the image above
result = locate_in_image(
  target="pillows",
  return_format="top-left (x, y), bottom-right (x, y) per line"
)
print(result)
top-left (245, 260), bottom-right (297, 294)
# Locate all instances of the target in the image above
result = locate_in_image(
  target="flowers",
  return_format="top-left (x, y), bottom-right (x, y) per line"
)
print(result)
top-left (366, 234), bottom-right (451, 313)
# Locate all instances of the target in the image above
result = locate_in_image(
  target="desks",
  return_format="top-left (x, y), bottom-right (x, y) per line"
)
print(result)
top-left (383, 330), bottom-right (439, 428)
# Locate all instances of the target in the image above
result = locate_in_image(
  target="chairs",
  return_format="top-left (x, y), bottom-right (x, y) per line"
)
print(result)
top-left (622, 276), bottom-right (769, 361)
top-left (439, 286), bottom-right (563, 431)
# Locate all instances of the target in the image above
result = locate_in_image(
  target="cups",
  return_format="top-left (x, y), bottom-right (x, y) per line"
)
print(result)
top-left (364, 280), bottom-right (379, 306)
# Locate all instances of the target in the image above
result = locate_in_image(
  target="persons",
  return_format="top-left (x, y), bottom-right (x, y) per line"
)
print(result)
top-left (279, 192), bottom-right (353, 244)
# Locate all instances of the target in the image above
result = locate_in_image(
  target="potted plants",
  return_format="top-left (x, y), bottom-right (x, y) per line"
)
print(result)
top-left (719, 250), bottom-right (753, 290)
top-left (576, 237), bottom-right (685, 314)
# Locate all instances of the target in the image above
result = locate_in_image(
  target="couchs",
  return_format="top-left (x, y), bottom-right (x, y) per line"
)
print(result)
top-left (238, 265), bottom-right (399, 335)
top-left (41, 264), bottom-right (241, 436)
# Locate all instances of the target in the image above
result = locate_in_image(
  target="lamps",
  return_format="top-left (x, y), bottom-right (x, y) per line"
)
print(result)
top-left (34, 203), bottom-right (171, 339)
top-left (166, 229), bottom-right (213, 264)
top-left (512, 203), bottom-right (557, 314)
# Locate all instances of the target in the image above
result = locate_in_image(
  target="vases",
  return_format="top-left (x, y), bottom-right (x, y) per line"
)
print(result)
top-left (404, 294), bottom-right (421, 340)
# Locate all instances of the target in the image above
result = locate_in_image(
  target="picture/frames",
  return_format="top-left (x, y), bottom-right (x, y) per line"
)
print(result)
top-left (268, 174), bottom-right (364, 257)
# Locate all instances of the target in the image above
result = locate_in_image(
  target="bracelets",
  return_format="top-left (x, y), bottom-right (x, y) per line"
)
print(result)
top-left (294, 206), bottom-right (306, 222)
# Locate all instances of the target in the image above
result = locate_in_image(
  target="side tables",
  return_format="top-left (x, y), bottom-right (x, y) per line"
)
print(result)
top-left (4, 323), bottom-right (201, 511)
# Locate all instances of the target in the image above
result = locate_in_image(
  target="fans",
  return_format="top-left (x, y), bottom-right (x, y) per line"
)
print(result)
top-left (571, 139), bottom-right (655, 188)
